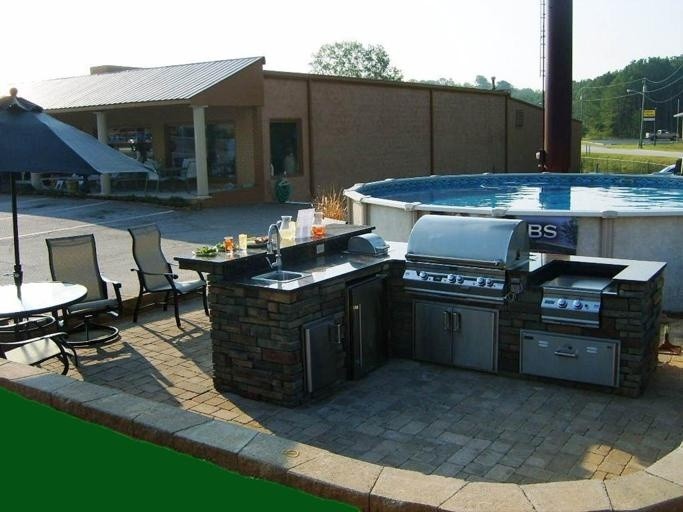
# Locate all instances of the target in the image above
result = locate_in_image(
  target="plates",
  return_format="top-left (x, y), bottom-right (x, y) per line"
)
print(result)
top-left (247, 240), bottom-right (273, 247)
top-left (214, 246), bottom-right (238, 251)
top-left (192, 249), bottom-right (218, 255)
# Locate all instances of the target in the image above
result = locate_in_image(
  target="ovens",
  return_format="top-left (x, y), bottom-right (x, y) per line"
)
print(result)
top-left (402, 214), bottom-right (530, 302)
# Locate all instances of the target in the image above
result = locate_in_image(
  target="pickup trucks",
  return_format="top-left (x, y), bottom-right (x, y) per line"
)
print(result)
top-left (646, 129), bottom-right (679, 140)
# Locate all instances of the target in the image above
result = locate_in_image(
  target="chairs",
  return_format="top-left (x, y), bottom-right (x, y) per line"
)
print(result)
top-left (46, 234), bottom-right (123, 346)
top-left (174, 162), bottom-right (198, 192)
top-left (175, 158), bottom-right (195, 191)
top-left (128, 223), bottom-right (209, 327)
top-left (144, 162), bottom-right (173, 195)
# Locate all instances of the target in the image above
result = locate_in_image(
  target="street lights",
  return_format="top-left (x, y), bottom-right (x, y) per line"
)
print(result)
top-left (627, 89), bottom-right (645, 149)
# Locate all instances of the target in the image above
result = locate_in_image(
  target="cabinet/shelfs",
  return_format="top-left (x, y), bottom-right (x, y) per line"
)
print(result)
top-left (175, 219), bottom-right (667, 406)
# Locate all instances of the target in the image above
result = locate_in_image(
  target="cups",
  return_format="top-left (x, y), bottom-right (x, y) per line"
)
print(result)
top-left (224, 236), bottom-right (233, 253)
top-left (276, 216), bottom-right (292, 239)
top-left (238, 234), bottom-right (247, 250)
top-left (312, 212), bottom-right (325, 234)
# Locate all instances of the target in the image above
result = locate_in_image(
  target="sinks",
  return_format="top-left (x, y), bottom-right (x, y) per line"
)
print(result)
top-left (250, 270), bottom-right (311, 282)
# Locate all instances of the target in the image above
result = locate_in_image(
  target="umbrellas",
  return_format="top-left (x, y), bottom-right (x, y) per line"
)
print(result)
top-left (1, 88), bottom-right (155, 283)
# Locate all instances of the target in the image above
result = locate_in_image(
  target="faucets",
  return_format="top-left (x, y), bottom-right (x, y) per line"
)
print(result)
top-left (264, 223), bottom-right (282, 273)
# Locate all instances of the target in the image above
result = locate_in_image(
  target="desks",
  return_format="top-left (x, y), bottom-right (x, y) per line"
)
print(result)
top-left (159, 167), bottom-right (186, 191)
top-left (0, 282), bottom-right (90, 376)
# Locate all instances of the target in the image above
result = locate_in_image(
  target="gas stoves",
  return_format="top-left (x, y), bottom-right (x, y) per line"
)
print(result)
top-left (538, 274), bottom-right (613, 328)
